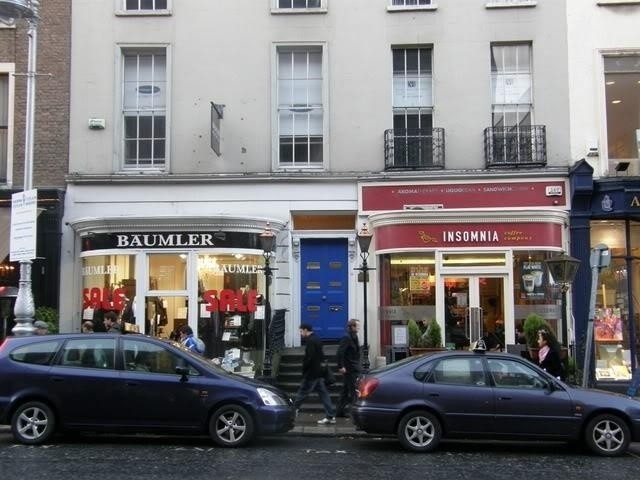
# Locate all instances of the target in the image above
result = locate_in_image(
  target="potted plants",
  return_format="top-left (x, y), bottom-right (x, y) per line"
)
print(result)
top-left (523, 314), bottom-right (560, 360)
top-left (407, 318), bottom-right (444, 355)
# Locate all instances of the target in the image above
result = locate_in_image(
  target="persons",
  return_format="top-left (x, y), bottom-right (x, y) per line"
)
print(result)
top-left (422, 317), bottom-right (429, 338)
top-left (33, 321), bottom-right (48, 335)
top-left (82, 321), bottom-right (94, 333)
top-left (336, 319), bottom-right (361, 419)
top-left (179, 325), bottom-right (204, 356)
top-left (537, 329), bottom-right (565, 382)
top-left (102, 311), bottom-right (124, 334)
top-left (294, 324), bottom-right (336, 425)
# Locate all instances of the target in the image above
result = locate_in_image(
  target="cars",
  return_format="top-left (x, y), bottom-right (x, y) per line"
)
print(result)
top-left (0, 333), bottom-right (295, 448)
top-left (351, 350), bottom-right (640, 457)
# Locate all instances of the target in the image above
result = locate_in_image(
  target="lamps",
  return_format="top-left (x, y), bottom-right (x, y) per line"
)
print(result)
top-left (614, 161), bottom-right (630, 175)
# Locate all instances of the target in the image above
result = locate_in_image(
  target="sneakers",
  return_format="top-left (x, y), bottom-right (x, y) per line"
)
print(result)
top-left (317, 416), bottom-right (336, 424)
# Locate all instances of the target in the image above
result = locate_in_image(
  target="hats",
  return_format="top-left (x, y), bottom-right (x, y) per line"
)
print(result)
top-left (35, 321), bottom-right (49, 329)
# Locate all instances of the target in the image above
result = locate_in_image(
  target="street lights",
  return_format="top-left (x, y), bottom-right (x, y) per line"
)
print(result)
top-left (254, 221), bottom-right (279, 384)
top-left (0, 0), bottom-right (43, 338)
top-left (353, 222), bottom-right (376, 377)
top-left (544, 251), bottom-right (580, 382)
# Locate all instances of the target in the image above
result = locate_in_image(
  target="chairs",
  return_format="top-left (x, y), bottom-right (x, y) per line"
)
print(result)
top-left (122, 348), bottom-right (158, 371)
top-left (472, 363), bottom-right (485, 385)
top-left (67, 347), bottom-right (103, 367)
